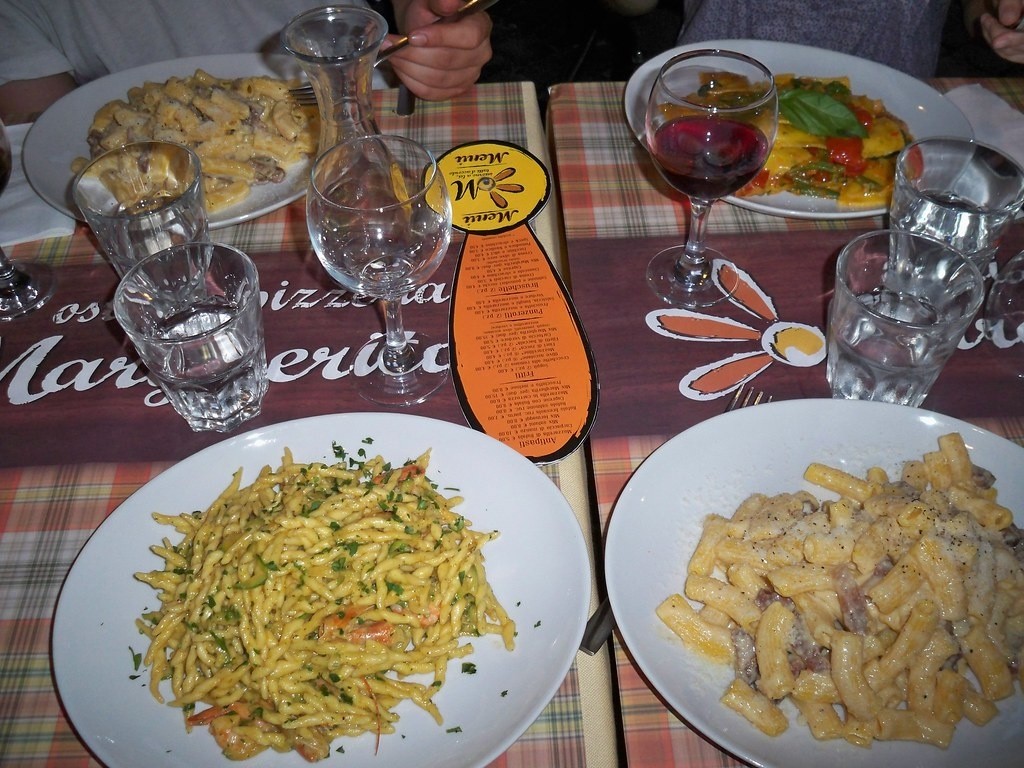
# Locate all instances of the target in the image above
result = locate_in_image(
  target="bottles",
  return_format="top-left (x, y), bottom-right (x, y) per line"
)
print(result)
top-left (278, 6), bottom-right (420, 241)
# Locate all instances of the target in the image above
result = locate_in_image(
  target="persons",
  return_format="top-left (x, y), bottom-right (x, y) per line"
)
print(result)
top-left (614, 0), bottom-right (1024, 83)
top-left (1, 0), bottom-right (495, 124)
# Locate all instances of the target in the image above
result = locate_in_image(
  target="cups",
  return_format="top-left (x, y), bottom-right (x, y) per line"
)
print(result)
top-left (113, 243), bottom-right (268, 432)
top-left (71, 140), bottom-right (211, 299)
top-left (887, 136), bottom-right (1024, 300)
top-left (823, 230), bottom-right (984, 405)
top-left (984, 251), bottom-right (1024, 376)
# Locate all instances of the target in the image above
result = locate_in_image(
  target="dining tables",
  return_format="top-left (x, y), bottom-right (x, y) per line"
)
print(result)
top-left (0, 79), bottom-right (625, 768)
top-left (544, 76), bottom-right (1024, 768)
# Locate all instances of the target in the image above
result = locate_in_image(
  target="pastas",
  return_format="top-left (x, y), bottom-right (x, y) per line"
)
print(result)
top-left (71, 66), bottom-right (321, 211)
top-left (654, 430), bottom-right (1024, 748)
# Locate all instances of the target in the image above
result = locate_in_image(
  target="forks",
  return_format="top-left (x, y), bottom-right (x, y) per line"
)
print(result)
top-left (581, 383), bottom-right (773, 656)
top-left (287, 0), bottom-right (498, 107)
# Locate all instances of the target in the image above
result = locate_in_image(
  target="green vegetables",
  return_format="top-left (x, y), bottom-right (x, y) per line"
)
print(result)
top-left (127, 436), bottom-right (510, 755)
top-left (721, 88), bottom-right (867, 140)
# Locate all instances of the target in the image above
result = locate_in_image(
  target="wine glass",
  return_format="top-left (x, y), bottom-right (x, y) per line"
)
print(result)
top-left (0, 123), bottom-right (53, 320)
top-left (644, 49), bottom-right (779, 308)
top-left (305, 134), bottom-right (451, 405)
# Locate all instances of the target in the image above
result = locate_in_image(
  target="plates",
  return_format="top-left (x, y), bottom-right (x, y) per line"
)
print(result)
top-left (48, 412), bottom-right (591, 768)
top-left (23, 53), bottom-right (359, 229)
top-left (605, 399), bottom-right (1024, 768)
top-left (624, 39), bottom-right (975, 221)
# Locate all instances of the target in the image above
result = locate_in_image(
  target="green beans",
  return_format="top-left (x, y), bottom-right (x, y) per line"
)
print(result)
top-left (785, 161), bottom-right (882, 199)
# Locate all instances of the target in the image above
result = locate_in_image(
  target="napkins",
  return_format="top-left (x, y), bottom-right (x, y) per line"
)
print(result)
top-left (0, 121), bottom-right (76, 248)
top-left (942, 83), bottom-right (1024, 221)
